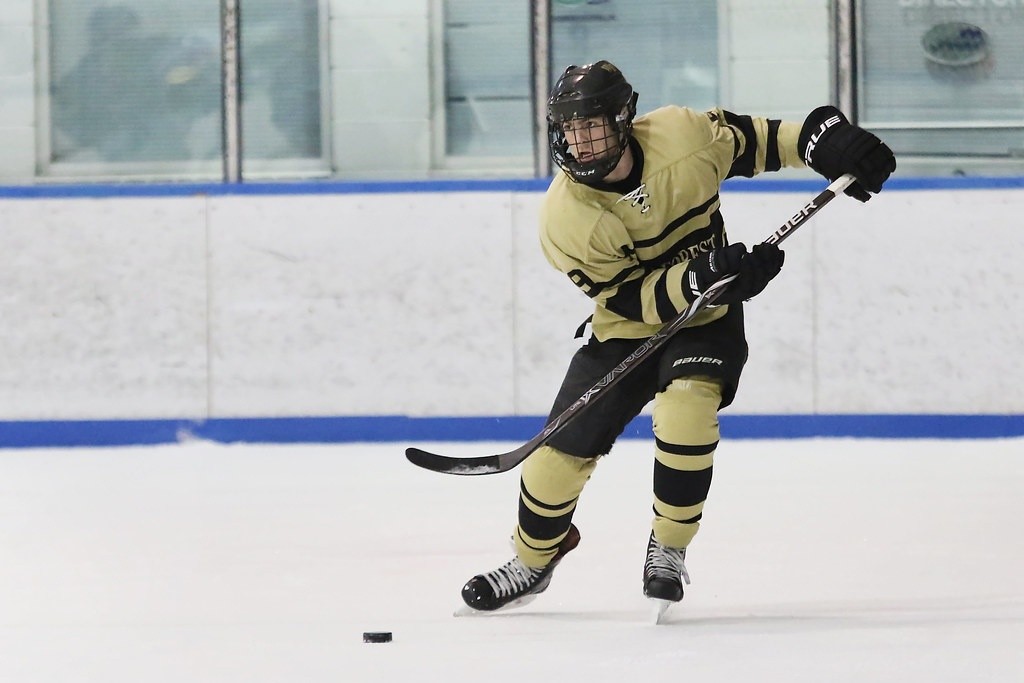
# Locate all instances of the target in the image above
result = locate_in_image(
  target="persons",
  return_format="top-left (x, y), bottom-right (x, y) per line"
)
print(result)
top-left (452, 60), bottom-right (897, 626)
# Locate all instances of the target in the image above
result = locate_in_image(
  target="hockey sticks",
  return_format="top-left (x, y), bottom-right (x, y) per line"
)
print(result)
top-left (401, 172), bottom-right (860, 479)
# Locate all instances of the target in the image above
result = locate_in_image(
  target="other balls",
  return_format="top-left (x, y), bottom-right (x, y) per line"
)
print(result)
top-left (361, 630), bottom-right (393, 644)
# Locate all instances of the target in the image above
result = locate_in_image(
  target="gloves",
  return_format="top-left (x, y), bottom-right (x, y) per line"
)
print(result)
top-left (680, 241), bottom-right (786, 311)
top-left (797, 105), bottom-right (896, 203)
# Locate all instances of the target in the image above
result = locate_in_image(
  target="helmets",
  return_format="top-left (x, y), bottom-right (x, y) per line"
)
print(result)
top-left (545, 59), bottom-right (638, 185)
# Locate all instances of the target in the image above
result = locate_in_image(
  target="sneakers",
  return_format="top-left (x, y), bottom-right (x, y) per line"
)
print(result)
top-left (453, 523), bottom-right (581, 617)
top-left (636, 528), bottom-right (691, 625)
